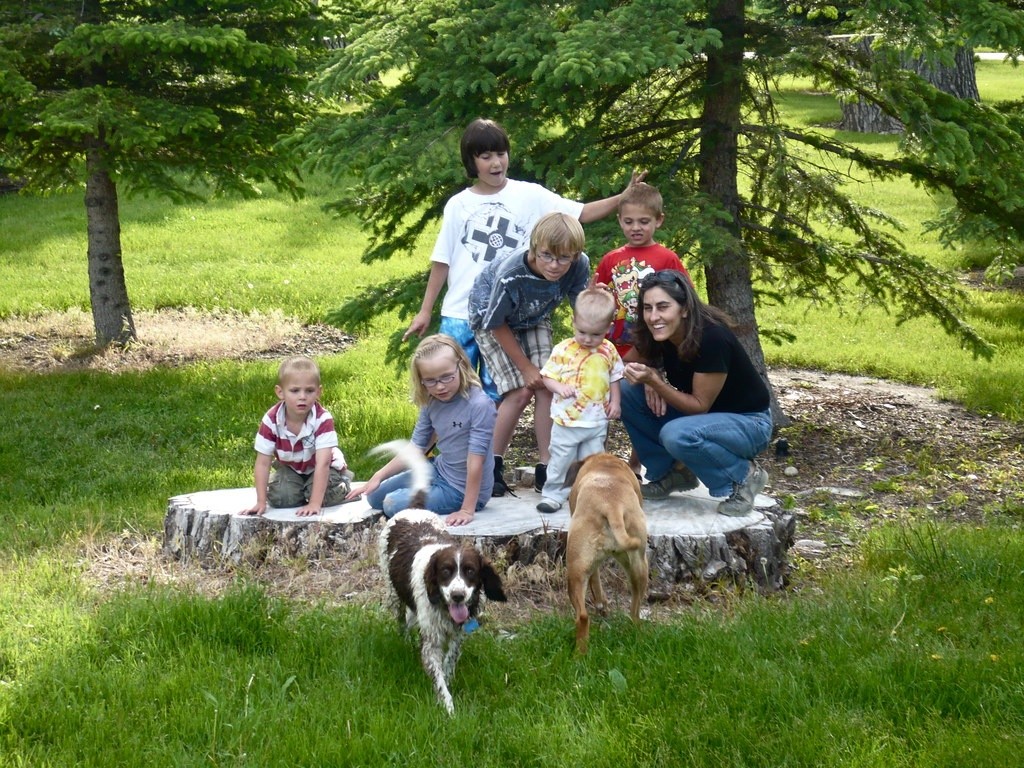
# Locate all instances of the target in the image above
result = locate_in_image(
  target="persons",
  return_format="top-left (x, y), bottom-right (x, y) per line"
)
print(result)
top-left (346, 118), bottom-right (775, 526)
top-left (240, 356), bottom-right (363, 517)
top-left (536, 286), bottom-right (629, 511)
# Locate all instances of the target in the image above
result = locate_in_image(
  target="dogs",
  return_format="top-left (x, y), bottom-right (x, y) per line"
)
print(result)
top-left (558, 452), bottom-right (650, 664)
top-left (366, 438), bottom-right (509, 722)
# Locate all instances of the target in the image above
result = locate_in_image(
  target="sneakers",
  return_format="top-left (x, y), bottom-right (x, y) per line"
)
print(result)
top-left (639, 465), bottom-right (700, 499)
top-left (718, 458), bottom-right (768, 517)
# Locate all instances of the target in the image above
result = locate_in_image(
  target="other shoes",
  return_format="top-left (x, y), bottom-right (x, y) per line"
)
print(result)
top-left (340, 468), bottom-right (354, 484)
top-left (492, 454), bottom-right (521, 500)
top-left (533, 464), bottom-right (548, 493)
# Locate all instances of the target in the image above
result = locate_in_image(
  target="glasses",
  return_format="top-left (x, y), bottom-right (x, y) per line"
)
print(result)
top-left (421, 362), bottom-right (461, 389)
top-left (642, 270), bottom-right (681, 286)
top-left (535, 249), bottom-right (574, 266)
